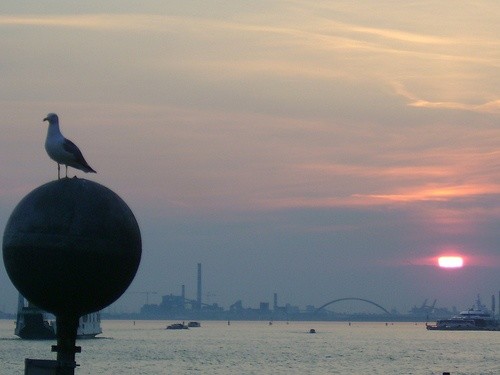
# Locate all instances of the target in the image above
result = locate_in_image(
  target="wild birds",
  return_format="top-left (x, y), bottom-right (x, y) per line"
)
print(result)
top-left (43, 112), bottom-right (97, 180)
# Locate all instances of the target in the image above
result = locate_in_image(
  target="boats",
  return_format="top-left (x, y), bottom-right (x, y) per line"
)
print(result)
top-left (12, 288), bottom-right (103, 342)
top-left (309, 328), bottom-right (316, 333)
top-left (188, 321), bottom-right (201, 327)
top-left (165, 323), bottom-right (189, 329)
top-left (425, 307), bottom-right (500, 331)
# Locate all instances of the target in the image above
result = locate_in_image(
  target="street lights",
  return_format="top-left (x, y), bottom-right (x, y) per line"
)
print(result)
top-left (3, 175), bottom-right (142, 375)
